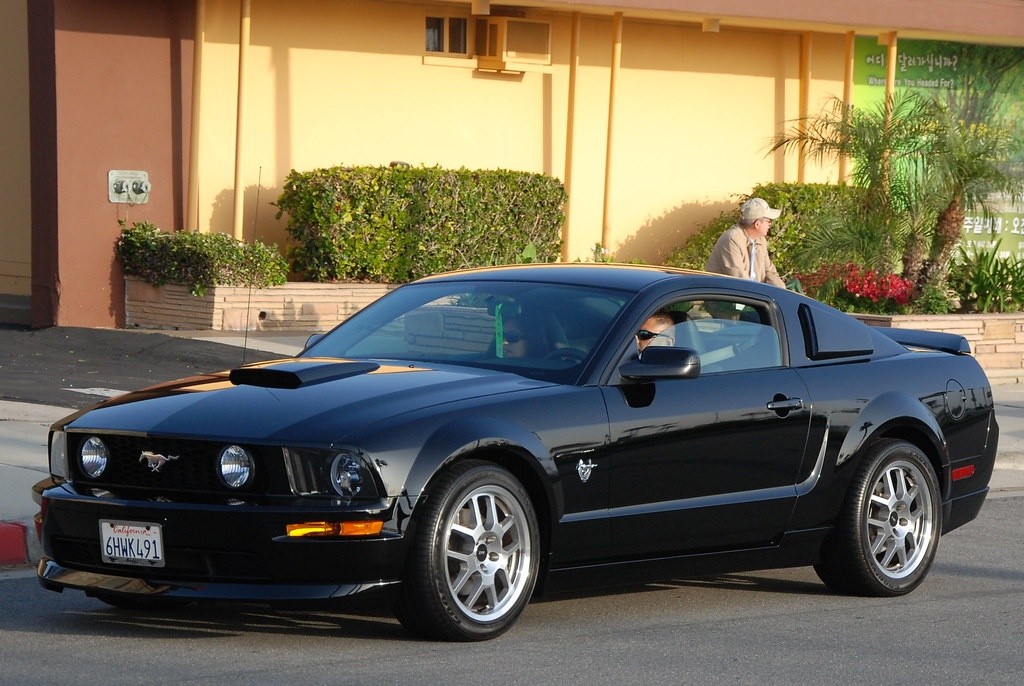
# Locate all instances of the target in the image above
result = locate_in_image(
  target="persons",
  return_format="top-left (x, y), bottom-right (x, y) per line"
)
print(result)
top-left (487, 315), bottom-right (538, 358)
top-left (634, 313), bottom-right (676, 362)
top-left (703, 196), bottom-right (788, 289)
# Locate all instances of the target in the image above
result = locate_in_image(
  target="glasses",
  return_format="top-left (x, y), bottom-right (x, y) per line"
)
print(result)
top-left (632, 328), bottom-right (671, 340)
top-left (502, 330), bottom-right (534, 342)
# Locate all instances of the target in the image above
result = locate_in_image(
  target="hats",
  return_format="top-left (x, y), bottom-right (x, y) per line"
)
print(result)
top-left (739, 197), bottom-right (781, 221)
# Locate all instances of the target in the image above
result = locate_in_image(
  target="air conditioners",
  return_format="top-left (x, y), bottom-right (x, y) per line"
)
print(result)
top-left (487, 16), bottom-right (553, 65)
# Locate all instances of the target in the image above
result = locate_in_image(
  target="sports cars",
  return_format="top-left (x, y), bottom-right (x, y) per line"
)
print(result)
top-left (24, 260), bottom-right (1000, 643)
